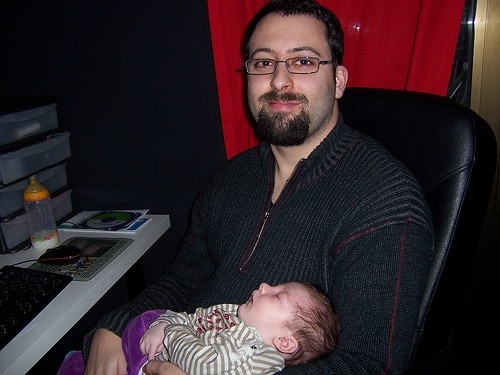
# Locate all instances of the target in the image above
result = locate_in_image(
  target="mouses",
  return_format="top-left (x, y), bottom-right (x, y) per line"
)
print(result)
top-left (38, 246), bottom-right (82, 264)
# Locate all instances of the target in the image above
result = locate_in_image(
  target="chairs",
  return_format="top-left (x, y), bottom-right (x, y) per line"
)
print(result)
top-left (268, 86), bottom-right (499, 375)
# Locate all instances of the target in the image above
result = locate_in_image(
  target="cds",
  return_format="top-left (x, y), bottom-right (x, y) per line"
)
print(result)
top-left (87, 212), bottom-right (131, 228)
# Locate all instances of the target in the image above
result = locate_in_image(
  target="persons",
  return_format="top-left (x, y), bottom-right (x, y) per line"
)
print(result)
top-left (57, 281), bottom-right (341, 375)
top-left (82, 0), bottom-right (435, 375)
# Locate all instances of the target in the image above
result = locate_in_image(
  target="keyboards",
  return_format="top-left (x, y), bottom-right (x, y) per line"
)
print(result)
top-left (0, 265), bottom-right (73, 351)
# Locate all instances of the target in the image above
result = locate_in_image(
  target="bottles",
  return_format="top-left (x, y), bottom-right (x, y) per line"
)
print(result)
top-left (23, 175), bottom-right (60, 251)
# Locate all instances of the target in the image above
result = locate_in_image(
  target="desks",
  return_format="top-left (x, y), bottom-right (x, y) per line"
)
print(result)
top-left (0, 209), bottom-right (172, 375)
